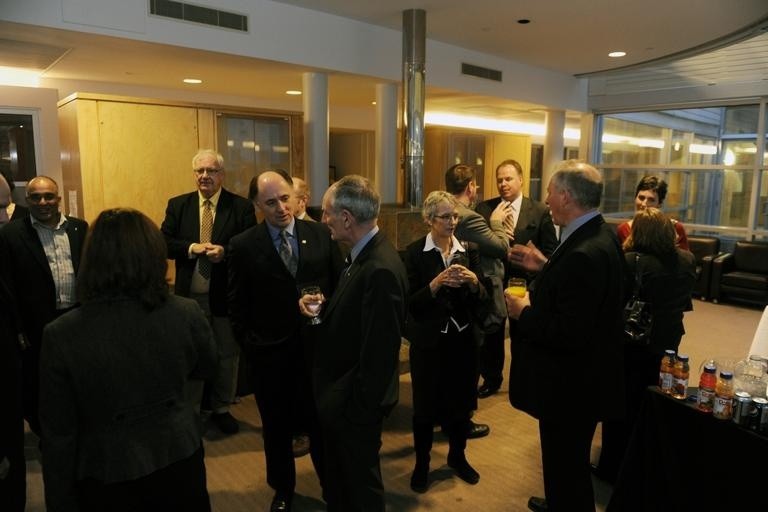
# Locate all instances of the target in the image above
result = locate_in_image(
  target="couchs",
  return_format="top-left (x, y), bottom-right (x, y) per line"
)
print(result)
top-left (684, 235), bottom-right (726, 301)
top-left (709, 240), bottom-right (768, 308)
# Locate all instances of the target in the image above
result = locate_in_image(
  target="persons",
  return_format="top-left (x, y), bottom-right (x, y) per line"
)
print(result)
top-left (0, 175), bottom-right (89, 438)
top-left (589, 206), bottom-right (697, 486)
top-left (503, 161), bottom-right (631, 512)
top-left (474, 159), bottom-right (558, 398)
top-left (617, 175), bottom-right (689, 258)
top-left (299, 175), bottom-right (410, 512)
top-left (228, 170), bottom-right (347, 512)
top-left (159, 149), bottom-right (257, 434)
top-left (444, 163), bottom-right (514, 440)
top-left (290, 177), bottom-right (317, 458)
top-left (0, 173), bottom-right (27, 512)
top-left (39, 206), bottom-right (219, 512)
top-left (405, 190), bottom-right (489, 493)
top-left (0, 166), bottom-right (28, 222)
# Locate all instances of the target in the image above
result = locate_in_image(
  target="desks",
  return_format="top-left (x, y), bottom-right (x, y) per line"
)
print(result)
top-left (643, 385), bottom-right (768, 512)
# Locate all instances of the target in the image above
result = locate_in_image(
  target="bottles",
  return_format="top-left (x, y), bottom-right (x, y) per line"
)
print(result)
top-left (746, 397), bottom-right (768, 432)
top-left (731, 391), bottom-right (751, 424)
top-left (696, 365), bottom-right (716, 412)
top-left (659, 350), bottom-right (675, 394)
top-left (671, 355), bottom-right (689, 400)
top-left (712, 371), bottom-right (734, 420)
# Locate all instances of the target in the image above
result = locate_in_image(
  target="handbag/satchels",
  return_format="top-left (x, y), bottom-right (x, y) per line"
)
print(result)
top-left (622, 251), bottom-right (656, 346)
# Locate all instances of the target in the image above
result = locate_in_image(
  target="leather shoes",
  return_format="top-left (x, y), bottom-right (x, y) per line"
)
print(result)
top-left (270, 494), bottom-right (290, 512)
top-left (293, 438), bottom-right (311, 456)
top-left (467, 420), bottom-right (489, 438)
top-left (209, 412), bottom-right (238, 433)
top-left (527, 495), bottom-right (546, 511)
top-left (447, 454), bottom-right (480, 483)
top-left (479, 381), bottom-right (498, 398)
top-left (411, 463), bottom-right (429, 492)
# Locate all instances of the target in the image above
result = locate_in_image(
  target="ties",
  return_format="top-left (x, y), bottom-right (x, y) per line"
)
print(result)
top-left (279, 230), bottom-right (298, 277)
top-left (503, 203), bottom-right (513, 232)
top-left (198, 201), bottom-right (214, 279)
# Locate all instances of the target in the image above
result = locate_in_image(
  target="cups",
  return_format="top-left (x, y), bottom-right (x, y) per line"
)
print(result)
top-left (302, 287), bottom-right (323, 324)
top-left (446, 254), bottom-right (460, 280)
top-left (508, 278), bottom-right (527, 297)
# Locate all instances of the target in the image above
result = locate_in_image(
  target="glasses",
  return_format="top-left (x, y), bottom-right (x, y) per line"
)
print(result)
top-left (433, 213), bottom-right (458, 220)
